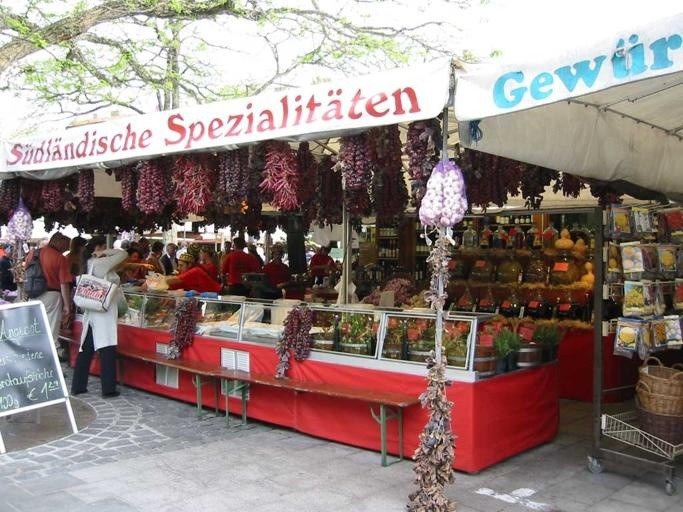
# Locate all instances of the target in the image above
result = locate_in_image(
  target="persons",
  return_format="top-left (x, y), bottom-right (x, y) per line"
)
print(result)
top-left (23, 231), bottom-right (70, 381)
top-left (0, 242), bottom-right (20, 297)
top-left (65, 230), bottom-right (338, 296)
top-left (70, 235), bottom-right (129, 397)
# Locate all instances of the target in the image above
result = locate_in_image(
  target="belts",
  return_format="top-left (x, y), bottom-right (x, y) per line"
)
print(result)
top-left (46, 288), bottom-right (61, 292)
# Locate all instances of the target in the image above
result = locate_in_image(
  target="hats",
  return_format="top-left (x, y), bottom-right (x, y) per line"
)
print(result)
top-left (178, 253), bottom-right (195, 265)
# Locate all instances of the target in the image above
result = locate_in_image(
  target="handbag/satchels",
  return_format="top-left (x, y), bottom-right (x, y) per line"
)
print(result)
top-left (72, 274), bottom-right (118, 314)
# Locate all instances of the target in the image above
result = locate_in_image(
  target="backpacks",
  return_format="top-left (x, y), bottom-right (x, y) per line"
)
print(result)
top-left (22, 247), bottom-right (47, 298)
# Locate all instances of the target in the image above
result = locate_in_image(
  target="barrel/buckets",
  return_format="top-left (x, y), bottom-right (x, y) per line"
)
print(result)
top-left (270, 299), bottom-right (301, 327)
top-left (478, 225), bottom-right (493, 249)
top-left (526, 224), bottom-right (541, 250)
top-left (461, 225), bottom-right (477, 248)
top-left (492, 225), bottom-right (508, 249)
top-left (508, 225), bottom-right (525, 250)
top-left (542, 222), bottom-right (559, 249)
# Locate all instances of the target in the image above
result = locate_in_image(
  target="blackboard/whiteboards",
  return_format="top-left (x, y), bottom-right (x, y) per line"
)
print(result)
top-left (0, 300), bottom-right (69, 417)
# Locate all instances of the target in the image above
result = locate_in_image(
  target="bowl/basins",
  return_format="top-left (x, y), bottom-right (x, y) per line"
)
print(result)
top-left (305, 340), bottom-right (541, 376)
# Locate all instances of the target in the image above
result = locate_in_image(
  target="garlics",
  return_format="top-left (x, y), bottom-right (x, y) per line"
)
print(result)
top-left (419, 170), bottom-right (468, 226)
top-left (7, 209), bottom-right (34, 245)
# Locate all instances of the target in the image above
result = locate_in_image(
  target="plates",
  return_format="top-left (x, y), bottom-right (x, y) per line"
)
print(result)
top-left (165, 291), bottom-right (246, 301)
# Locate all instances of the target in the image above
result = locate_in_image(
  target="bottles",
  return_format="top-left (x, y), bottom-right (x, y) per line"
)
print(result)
top-left (379, 225), bottom-right (433, 284)
top-left (452, 214), bottom-right (598, 318)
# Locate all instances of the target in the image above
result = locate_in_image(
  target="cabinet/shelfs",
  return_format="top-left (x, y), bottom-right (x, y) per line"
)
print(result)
top-left (371, 204), bottom-right (595, 283)
top-left (57, 282), bottom-right (563, 474)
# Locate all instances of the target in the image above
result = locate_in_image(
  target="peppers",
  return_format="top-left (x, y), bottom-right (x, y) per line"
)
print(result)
top-left (259, 142), bottom-right (300, 213)
top-left (168, 153), bottom-right (218, 216)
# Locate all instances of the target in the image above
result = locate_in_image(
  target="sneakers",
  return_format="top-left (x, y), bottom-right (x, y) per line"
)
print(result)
top-left (102, 391), bottom-right (120, 398)
top-left (71, 388), bottom-right (89, 396)
